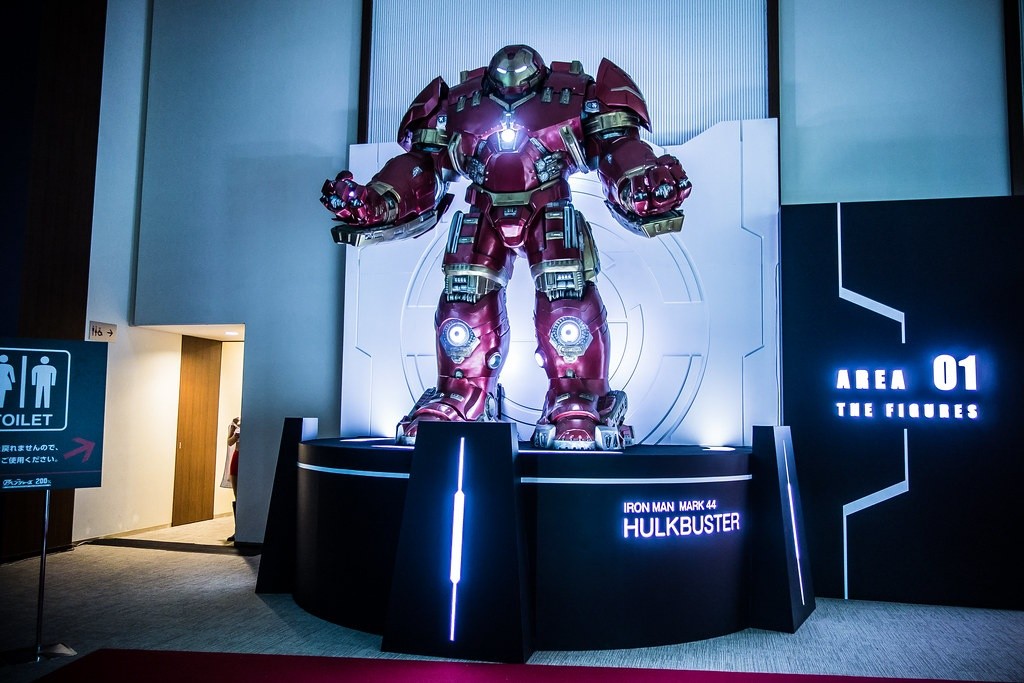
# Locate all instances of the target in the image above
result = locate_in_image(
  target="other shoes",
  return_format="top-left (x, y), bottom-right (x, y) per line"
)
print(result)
top-left (227, 534), bottom-right (235, 541)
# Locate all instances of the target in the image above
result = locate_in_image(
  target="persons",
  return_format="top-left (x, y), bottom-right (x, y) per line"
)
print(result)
top-left (227, 417), bottom-right (241, 542)
top-left (319, 45), bottom-right (693, 450)
